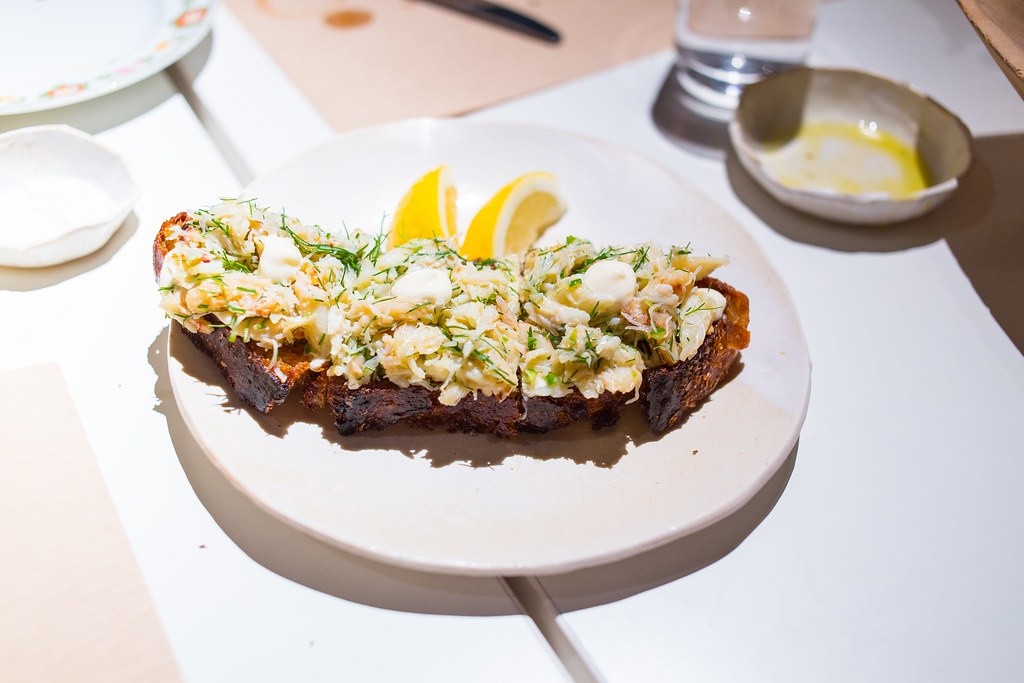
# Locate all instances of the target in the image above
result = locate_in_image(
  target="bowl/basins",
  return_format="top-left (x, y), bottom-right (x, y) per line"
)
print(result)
top-left (730, 67), bottom-right (976, 225)
top-left (0, 126), bottom-right (136, 267)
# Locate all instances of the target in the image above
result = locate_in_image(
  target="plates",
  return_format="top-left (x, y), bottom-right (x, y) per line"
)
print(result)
top-left (0, 0), bottom-right (217, 121)
top-left (165, 116), bottom-right (811, 580)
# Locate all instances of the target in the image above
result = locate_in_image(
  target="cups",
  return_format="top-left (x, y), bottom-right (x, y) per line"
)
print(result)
top-left (670, 0), bottom-right (817, 122)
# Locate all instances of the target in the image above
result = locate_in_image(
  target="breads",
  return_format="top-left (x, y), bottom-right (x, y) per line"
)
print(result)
top-left (151, 211), bottom-right (752, 441)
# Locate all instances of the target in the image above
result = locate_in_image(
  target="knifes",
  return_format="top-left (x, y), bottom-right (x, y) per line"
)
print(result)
top-left (421, 0), bottom-right (562, 45)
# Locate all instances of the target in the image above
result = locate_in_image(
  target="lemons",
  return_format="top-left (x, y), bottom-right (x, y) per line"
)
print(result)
top-left (385, 164), bottom-right (461, 249)
top-left (460, 172), bottom-right (566, 266)
